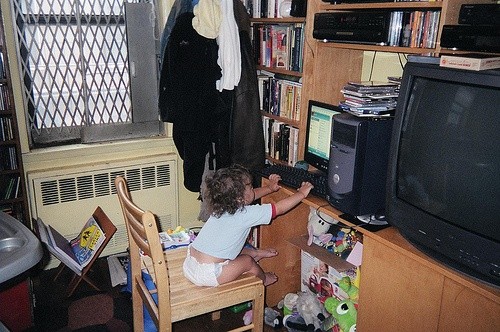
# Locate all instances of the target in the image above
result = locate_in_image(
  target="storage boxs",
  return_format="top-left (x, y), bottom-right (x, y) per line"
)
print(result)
top-left (285, 232), bottom-right (359, 295)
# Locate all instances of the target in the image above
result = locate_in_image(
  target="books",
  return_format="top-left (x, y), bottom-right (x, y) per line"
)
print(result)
top-left (244, 0), bottom-right (306, 168)
top-left (46, 214), bottom-right (107, 270)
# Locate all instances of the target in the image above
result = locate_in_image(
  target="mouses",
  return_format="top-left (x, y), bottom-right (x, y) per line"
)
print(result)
top-left (374, 207), bottom-right (386, 221)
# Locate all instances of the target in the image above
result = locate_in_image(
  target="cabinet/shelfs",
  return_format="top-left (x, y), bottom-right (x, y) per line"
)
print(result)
top-left (241, 0), bottom-right (500, 332)
top-left (0, 8), bottom-right (33, 230)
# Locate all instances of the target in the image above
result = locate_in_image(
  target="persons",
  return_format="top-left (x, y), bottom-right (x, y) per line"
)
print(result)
top-left (182, 165), bottom-right (313, 288)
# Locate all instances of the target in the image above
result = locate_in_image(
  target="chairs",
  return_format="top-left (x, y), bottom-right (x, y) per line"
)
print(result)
top-left (114, 176), bottom-right (265, 332)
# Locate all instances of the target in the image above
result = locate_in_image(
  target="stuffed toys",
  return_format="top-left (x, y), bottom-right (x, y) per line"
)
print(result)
top-left (244, 211), bottom-right (361, 332)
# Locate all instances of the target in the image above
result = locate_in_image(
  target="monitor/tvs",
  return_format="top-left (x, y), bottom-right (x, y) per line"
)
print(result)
top-left (385, 62), bottom-right (500, 289)
top-left (304, 100), bottom-right (345, 174)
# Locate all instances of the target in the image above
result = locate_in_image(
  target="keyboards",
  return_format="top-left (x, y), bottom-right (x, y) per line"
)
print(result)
top-left (257, 164), bottom-right (329, 200)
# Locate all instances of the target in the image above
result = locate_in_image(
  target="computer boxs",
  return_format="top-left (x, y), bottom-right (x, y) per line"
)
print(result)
top-left (327, 114), bottom-right (394, 216)
